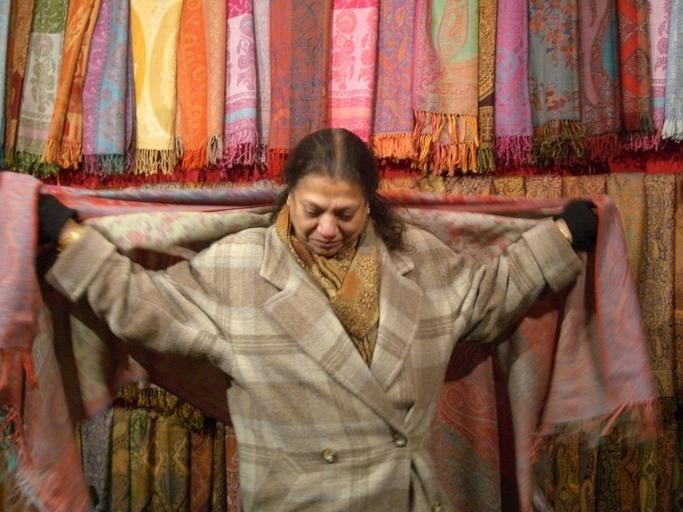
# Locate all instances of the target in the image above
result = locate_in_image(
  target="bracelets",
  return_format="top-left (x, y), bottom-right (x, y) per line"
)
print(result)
top-left (51, 222), bottom-right (92, 251)
top-left (555, 218), bottom-right (578, 247)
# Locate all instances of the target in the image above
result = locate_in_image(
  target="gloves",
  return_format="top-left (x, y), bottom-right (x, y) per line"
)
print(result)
top-left (37, 195), bottom-right (77, 243)
top-left (561, 200), bottom-right (597, 248)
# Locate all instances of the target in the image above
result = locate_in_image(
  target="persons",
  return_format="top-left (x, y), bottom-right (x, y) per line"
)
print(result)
top-left (39, 129), bottom-right (598, 511)
